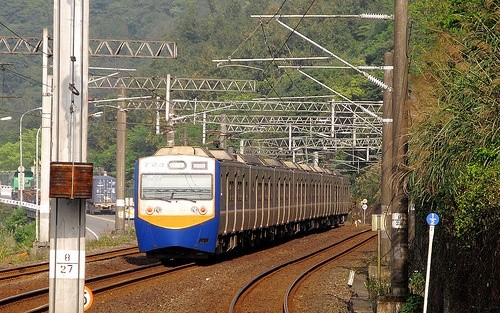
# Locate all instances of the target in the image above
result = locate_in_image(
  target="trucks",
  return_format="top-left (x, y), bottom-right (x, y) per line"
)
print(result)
top-left (86, 176), bottom-right (118, 215)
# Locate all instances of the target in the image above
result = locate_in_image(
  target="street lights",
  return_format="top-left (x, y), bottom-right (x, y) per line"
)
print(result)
top-left (18, 107), bottom-right (43, 197)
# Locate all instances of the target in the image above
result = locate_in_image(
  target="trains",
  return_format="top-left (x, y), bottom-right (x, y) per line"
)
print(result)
top-left (135, 144), bottom-right (352, 263)
